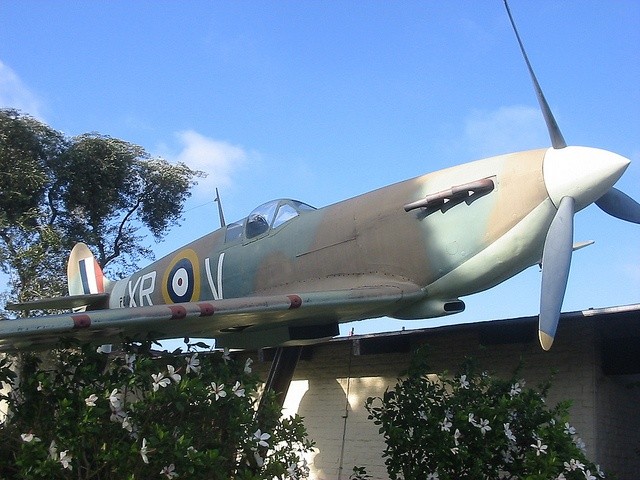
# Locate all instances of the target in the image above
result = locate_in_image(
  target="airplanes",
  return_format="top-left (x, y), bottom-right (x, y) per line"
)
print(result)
top-left (0, 1), bottom-right (640, 352)
